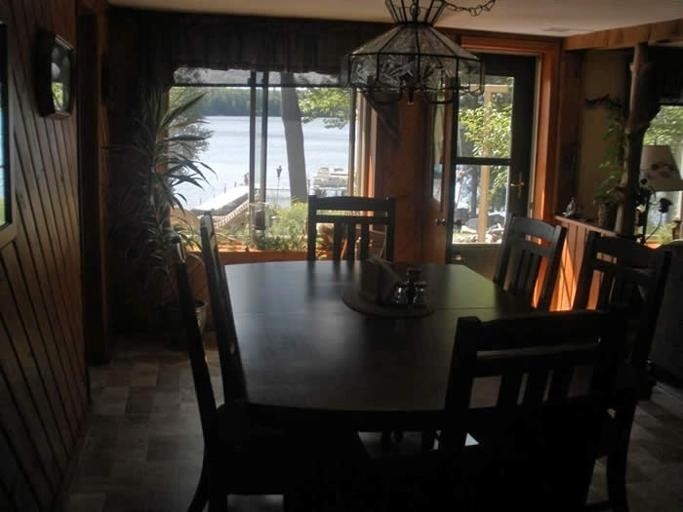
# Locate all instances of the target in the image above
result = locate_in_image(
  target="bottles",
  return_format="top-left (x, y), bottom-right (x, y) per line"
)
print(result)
top-left (391, 267), bottom-right (428, 309)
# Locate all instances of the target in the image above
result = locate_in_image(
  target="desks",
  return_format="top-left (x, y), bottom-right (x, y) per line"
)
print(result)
top-left (224, 259), bottom-right (593, 475)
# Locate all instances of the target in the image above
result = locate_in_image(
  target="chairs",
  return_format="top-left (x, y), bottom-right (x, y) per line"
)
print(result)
top-left (199, 211), bottom-right (246, 407)
top-left (305, 195), bottom-right (399, 261)
top-left (568, 229), bottom-right (669, 401)
top-left (170, 228), bottom-right (375, 510)
top-left (489, 212), bottom-right (568, 315)
top-left (411, 281), bottom-right (647, 511)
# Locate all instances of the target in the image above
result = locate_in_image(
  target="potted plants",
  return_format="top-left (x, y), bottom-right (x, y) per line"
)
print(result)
top-left (585, 95), bottom-right (635, 231)
top-left (100, 82), bottom-right (209, 349)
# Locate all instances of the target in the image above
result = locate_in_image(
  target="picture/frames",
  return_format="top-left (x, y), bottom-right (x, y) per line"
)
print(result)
top-left (31, 29), bottom-right (77, 119)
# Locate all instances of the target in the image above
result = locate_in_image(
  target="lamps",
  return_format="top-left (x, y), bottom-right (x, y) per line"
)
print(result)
top-left (638, 146), bottom-right (683, 245)
top-left (338, 1), bottom-right (496, 109)
